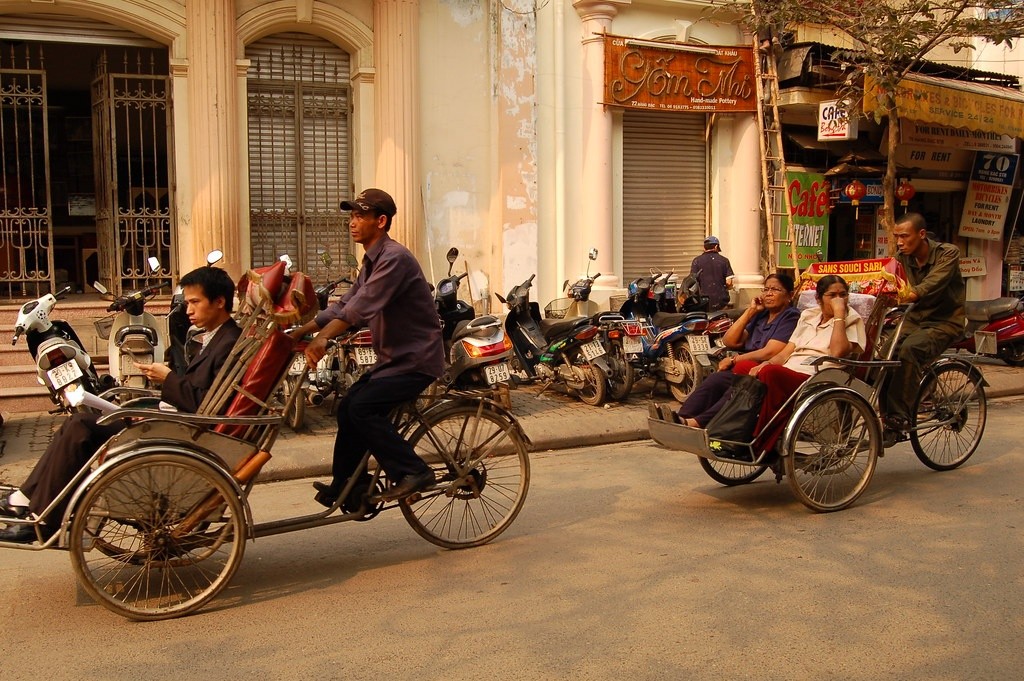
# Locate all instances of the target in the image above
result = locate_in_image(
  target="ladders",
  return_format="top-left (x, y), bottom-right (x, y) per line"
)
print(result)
top-left (752, 0), bottom-right (800, 287)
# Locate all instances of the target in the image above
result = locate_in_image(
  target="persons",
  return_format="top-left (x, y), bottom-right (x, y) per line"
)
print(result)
top-left (713, 275), bottom-right (866, 458)
top-left (648, 273), bottom-right (801, 428)
top-left (286, 189), bottom-right (446, 502)
top-left (0, 266), bottom-right (246, 544)
top-left (877, 214), bottom-right (966, 448)
top-left (691, 236), bottom-right (735, 313)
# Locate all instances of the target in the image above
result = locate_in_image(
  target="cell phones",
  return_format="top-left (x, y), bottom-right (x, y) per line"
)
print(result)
top-left (125, 348), bottom-right (140, 363)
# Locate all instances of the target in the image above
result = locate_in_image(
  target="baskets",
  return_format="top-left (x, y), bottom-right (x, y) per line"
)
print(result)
top-left (94, 313), bottom-right (121, 340)
top-left (544, 298), bottom-right (576, 318)
top-left (610, 295), bottom-right (630, 312)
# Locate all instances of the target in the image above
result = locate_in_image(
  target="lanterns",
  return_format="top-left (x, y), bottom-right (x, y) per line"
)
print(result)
top-left (894, 180), bottom-right (915, 214)
top-left (845, 180), bottom-right (866, 220)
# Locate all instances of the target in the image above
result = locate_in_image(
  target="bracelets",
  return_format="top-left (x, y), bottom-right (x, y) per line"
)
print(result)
top-left (731, 353), bottom-right (738, 365)
top-left (833, 318), bottom-right (846, 323)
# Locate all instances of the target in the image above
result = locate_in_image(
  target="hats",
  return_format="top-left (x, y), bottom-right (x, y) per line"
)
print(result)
top-left (340, 188), bottom-right (397, 218)
top-left (704, 236), bottom-right (722, 252)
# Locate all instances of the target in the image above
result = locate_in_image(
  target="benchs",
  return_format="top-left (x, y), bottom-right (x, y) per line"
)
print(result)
top-left (91, 261), bottom-right (317, 504)
top-left (729, 287), bottom-right (886, 445)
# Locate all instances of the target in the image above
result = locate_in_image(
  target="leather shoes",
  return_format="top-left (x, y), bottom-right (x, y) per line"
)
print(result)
top-left (0, 523), bottom-right (59, 543)
top-left (0, 496), bottom-right (30, 519)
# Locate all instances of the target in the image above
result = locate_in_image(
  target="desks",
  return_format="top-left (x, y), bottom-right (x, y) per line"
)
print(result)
top-left (23, 225), bottom-right (121, 286)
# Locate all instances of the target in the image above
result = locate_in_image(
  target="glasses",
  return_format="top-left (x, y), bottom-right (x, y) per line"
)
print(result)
top-left (760, 286), bottom-right (788, 295)
top-left (823, 292), bottom-right (849, 298)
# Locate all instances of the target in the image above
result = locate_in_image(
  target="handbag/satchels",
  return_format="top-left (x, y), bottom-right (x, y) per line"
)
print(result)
top-left (704, 375), bottom-right (768, 452)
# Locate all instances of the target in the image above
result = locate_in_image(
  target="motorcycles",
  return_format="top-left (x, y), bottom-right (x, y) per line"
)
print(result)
top-left (495, 273), bottom-right (614, 406)
top-left (544, 246), bottom-right (647, 402)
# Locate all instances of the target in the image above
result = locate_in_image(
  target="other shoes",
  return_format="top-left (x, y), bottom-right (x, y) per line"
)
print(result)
top-left (882, 417), bottom-right (912, 430)
top-left (312, 481), bottom-right (340, 498)
top-left (662, 404), bottom-right (689, 425)
top-left (648, 401), bottom-right (663, 420)
top-left (379, 467), bottom-right (436, 501)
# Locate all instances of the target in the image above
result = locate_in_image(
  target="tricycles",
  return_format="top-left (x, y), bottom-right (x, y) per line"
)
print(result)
top-left (0, 322), bottom-right (535, 622)
top-left (646, 290), bottom-right (992, 513)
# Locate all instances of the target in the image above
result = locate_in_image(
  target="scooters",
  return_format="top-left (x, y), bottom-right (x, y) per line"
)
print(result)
top-left (646, 269), bottom-right (735, 381)
top-left (94, 256), bottom-right (169, 405)
top-left (309, 252), bottom-right (380, 420)
top-left (609, 267), bottom-right (711, 405)
top-left (165, 249), bottom-right (223, 376)
top-left (423, 247), bottom-right (518, 415)
top-left (238, 260), bottom-right (325, 430)
top-left (946, 297), bottom-right (1024, 369)
top-left (11, 285), bottom-right (116, 416)
